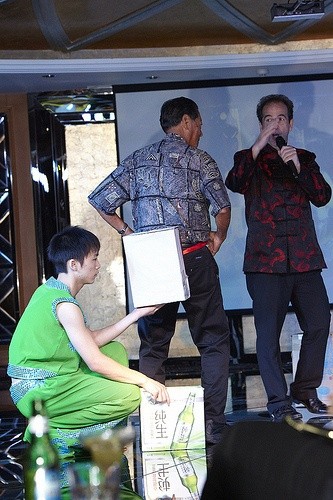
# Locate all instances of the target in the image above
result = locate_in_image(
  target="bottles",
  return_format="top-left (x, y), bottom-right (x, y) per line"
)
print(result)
top-left (170, 393), bottom-right (195, 450)
top-left (169, 451), bottom-right (200, 500)
top-left (24, 400), bottom-right (62, 500)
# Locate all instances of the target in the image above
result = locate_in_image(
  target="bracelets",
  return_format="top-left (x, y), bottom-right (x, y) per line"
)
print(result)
top-left (118, 223), bottom-right (128, 234)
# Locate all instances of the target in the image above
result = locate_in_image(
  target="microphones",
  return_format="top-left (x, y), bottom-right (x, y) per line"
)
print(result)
top-left (276, 135), bottom-right (299, 179)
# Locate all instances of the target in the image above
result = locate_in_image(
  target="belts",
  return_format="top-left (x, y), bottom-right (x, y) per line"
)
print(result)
top-left (182, 241), bottom-right (208, 256)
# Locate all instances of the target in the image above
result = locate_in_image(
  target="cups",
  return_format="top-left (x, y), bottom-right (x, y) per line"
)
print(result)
top-left (87, 429), bottom-right (121, 500)
top-left (68, 464), bottom-right (106, 500)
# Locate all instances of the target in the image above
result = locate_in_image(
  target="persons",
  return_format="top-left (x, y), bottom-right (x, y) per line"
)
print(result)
top-left (88, 97), bottom-right (231, 444)
top-left (7, 226), bottom-right (171, 457)
top-left (225, 94), bottom-right (331, 421)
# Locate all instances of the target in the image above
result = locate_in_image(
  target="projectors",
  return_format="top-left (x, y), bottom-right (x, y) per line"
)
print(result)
top-left (270, 1), bottom-right (325, 22)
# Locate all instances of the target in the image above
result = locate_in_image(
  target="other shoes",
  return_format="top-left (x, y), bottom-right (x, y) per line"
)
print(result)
top-left (206, 420), bottom-right (230, 444)
top-left (22, 426), bottom-right (74, 458)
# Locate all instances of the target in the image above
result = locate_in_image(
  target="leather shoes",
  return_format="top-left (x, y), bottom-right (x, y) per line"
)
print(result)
top-left (269, 406), bottom-right (303, 420)
top-left (291, 393), bottom-right (328, 414)
